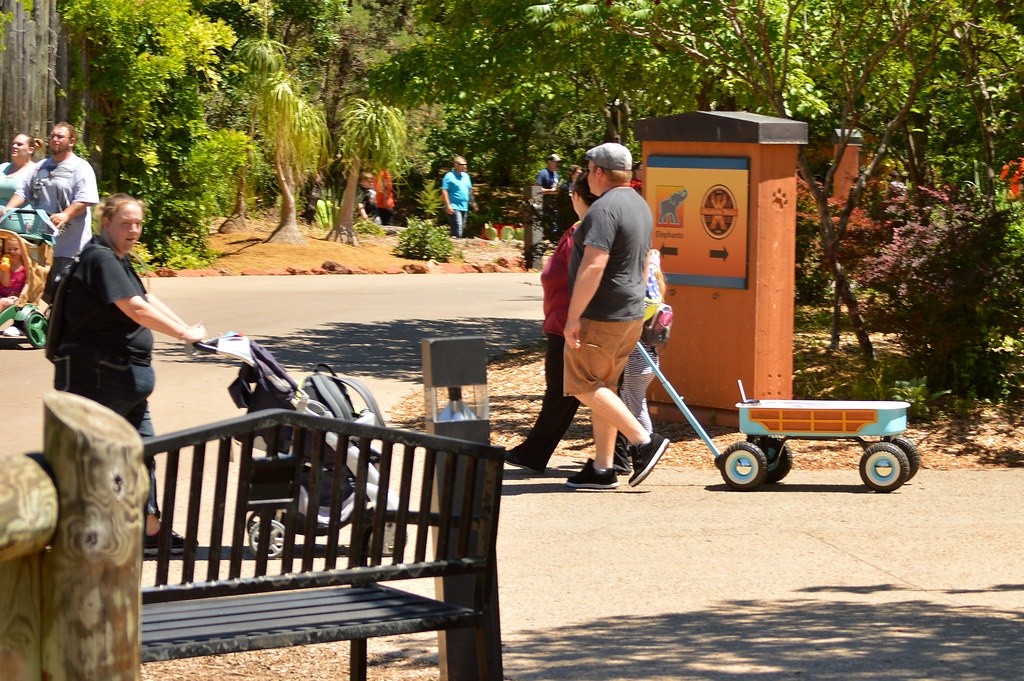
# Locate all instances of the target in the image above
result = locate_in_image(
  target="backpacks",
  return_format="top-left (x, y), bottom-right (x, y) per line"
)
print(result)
top-left (301, 363), bottom-right (385, 427)
top-left (641, 303), bottom-right (673, 357)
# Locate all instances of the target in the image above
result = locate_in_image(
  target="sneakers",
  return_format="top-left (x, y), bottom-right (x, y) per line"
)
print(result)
top-left (3, 324), bottom-right (26, 337)
top-left (144, 523), bottom-right (199, 554)
top-left (566, 458), bottom-right (619, 488)
top-left (628, 433), bottom-right (670, 487)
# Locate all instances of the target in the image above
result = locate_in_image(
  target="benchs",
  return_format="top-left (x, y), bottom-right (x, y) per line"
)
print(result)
top-left (134, 406), bottom-right (507, 681)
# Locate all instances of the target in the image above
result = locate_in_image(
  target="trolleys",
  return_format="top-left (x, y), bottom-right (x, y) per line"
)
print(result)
top-left (632, 340), bottom-right (920, 493)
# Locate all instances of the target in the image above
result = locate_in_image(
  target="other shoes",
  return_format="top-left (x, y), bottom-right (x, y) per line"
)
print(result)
top-left (504, 450), bottom-right (544, 474)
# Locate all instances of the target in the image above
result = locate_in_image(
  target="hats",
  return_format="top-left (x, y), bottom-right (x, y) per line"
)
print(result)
top-left (585, 143), bottom-right (632, 171)
top-left (548, 154), bottom-right (562, 162)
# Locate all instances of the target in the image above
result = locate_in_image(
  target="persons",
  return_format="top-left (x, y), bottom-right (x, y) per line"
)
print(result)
top-left (504, 142), bottom-right (669, 488)
top-left (441, 156), bottom-right (479, 238)
top-left (0, 121), bottom-right (99, 336)
top-left (358, 171), bottom-right (382, 225)
top-left (46, 193), bottom-right (209, 554)
top-left (374, 161), bottom-right (395, 225)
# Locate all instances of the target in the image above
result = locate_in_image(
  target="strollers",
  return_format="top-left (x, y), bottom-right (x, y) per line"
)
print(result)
top-left (0, 208), bottom-right (65, 349)
top-left (191, 329), bottom-right (408, 560)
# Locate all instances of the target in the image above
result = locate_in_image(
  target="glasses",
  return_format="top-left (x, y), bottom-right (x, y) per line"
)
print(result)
top-left (568, 190), bottom-right (575, 196)
